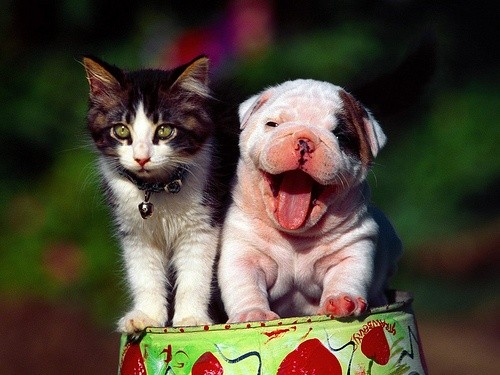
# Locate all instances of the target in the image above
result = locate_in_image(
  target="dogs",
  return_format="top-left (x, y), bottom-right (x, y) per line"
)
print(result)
top-left (217, 78), bottom-right (403, 324)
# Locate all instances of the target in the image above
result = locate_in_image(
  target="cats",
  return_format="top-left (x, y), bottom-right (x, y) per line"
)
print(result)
top-left (70, 53), bottom-right (235, 334)
